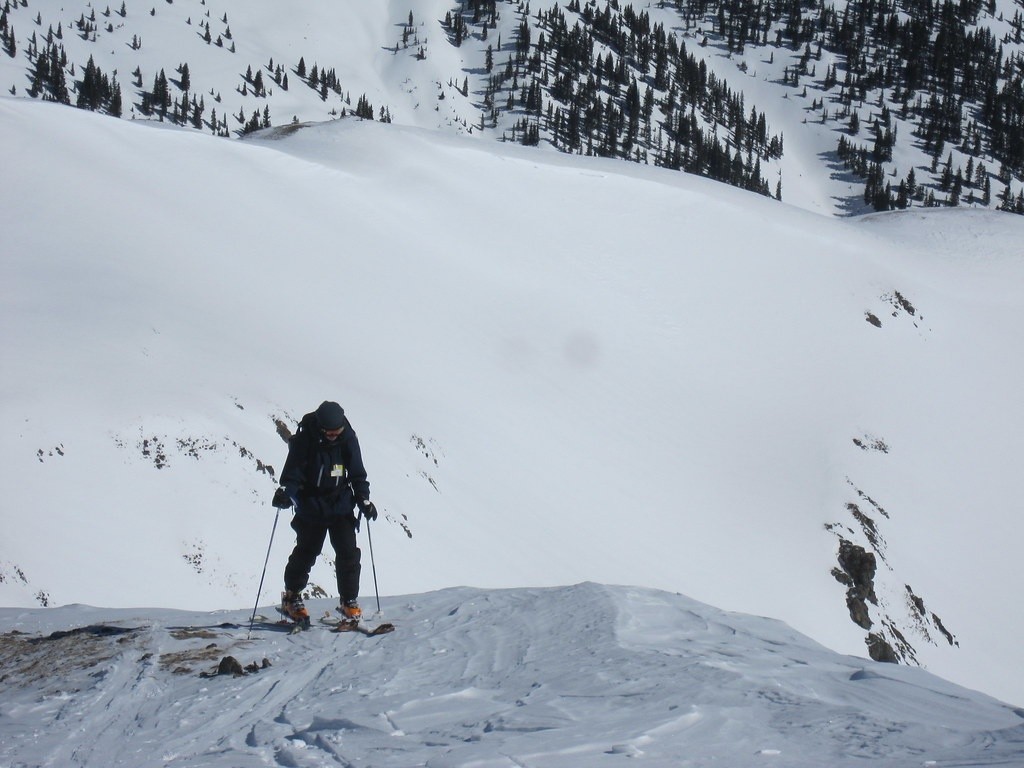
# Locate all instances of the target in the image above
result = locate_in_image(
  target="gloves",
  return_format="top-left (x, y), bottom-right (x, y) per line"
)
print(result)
top-left (359, 503), bottom-right (377, 522)
top-left (272, 488), bottom-right (294, 509)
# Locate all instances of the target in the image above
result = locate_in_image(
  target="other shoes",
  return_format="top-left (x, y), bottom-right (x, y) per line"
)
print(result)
top-left (337, 601), bottom-right (361, 619)
top-left (281, 596), bottom-right (308, 620)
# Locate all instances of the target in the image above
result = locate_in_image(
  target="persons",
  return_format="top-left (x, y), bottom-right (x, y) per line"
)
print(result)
top-left (272, 402), bottom-right (378, 624)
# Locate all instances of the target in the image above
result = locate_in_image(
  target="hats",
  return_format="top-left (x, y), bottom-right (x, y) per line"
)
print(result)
top-left (318, 400), bottom-right (344, 430)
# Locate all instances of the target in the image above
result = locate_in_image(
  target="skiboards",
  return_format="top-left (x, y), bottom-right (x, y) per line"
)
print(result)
top-left (249, 611), bottom-right (395, 637)
